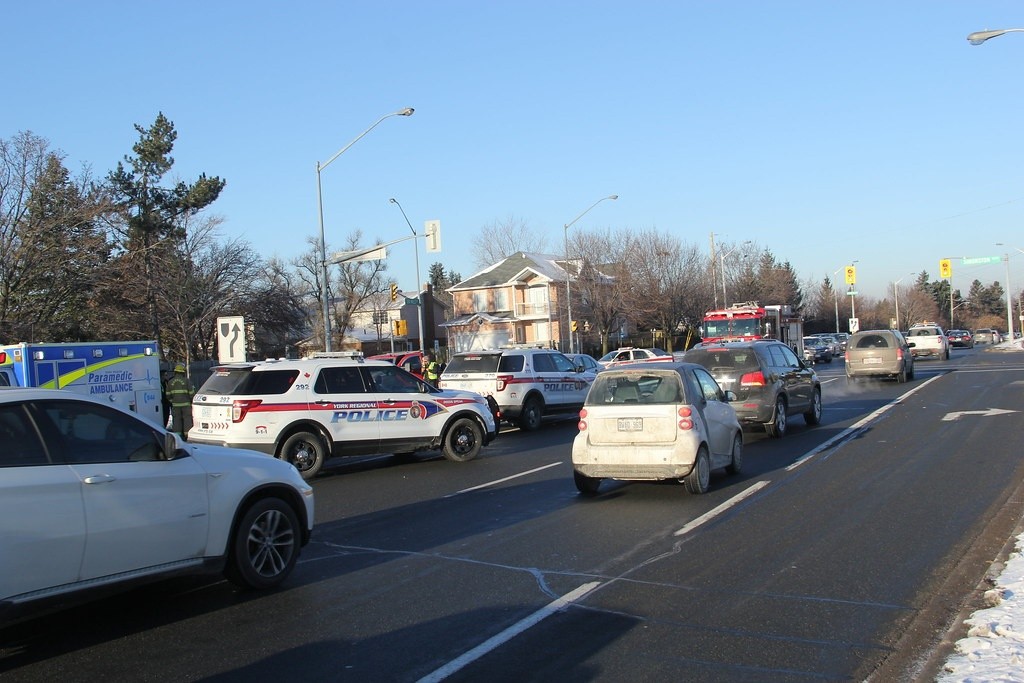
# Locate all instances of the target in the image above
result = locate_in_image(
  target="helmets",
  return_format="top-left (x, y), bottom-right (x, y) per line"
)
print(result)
top-left (174, 365), bottom-right (185, 372)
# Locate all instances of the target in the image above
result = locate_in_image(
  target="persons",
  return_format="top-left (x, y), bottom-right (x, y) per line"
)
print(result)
top-left (421, 356), bottom-right (439, 388)
top-left (160, 365), bottom-right (194, 440)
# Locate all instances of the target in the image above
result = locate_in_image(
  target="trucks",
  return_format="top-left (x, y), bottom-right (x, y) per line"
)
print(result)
top-left (0, 342), bottom-right (166, 430)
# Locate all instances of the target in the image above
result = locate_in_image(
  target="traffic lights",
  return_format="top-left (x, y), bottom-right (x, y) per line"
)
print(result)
top-left (940, 259), bottom-right (951, 278)
top-left (571, 320), bottom-right (578, 333)
top-left (391, 284), bottom-right (398, 302)
top-left (845, 266), bottom-right (856, 283)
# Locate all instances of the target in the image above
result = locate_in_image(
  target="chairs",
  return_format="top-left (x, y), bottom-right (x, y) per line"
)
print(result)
top-left (615, 384), bottom-right (643, 402)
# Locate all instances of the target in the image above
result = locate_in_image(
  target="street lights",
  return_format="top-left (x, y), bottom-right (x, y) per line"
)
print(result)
top-left (720, 241), bottom-right (751, 309)
top-left (317, 106), bottom-right (415, 352)
top-left (565, 196), bottom-right (620, 353)
top-left (895, 272), bottom-right (916, 330)
top-left (390, 198), bottom-right (425, 353)
top-left (951, 301), bottom-right (971, 329)
top-left (833, 259), bottom-right (861, 332)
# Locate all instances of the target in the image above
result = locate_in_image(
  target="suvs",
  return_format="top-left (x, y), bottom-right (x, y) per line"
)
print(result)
top-left (904, 322), bottom-right (950, 360)
top-left (844, 329), bottom-right (917, 382)
top-left (437, 346), bottom-right (601, 431)
top-left (0, 385), bottom-right (318, 612)
top-left (185, 352), bottom-right (502, 480)
top-left (683, 341), bottom-right (824, 436)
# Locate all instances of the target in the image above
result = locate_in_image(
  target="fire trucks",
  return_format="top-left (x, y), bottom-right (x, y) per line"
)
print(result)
top-left (701, 301), bottom-right (803, 360)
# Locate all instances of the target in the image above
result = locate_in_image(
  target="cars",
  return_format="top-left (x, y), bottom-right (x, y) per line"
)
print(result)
top-left (804, 333), bottom-right (849, 362)
top-left (596, 346), bottom-right (675, 365)
top-left (554, 354), bottom-right (605, 375)
top-left (1003, 332), bottom-right (1021, 339)
top-left (944, 330), bottom-right (974, 348)
top-left (973, 327), bottom-right (1004, 345)
top-left (572, 363), bottom-right (744, 494)
